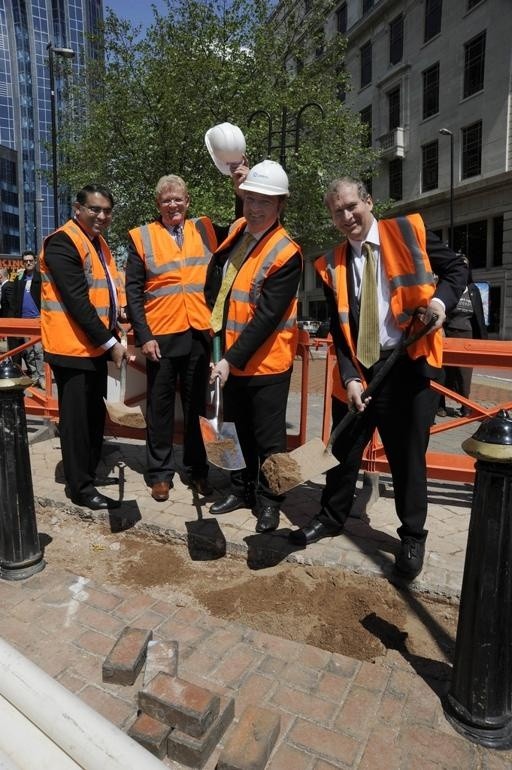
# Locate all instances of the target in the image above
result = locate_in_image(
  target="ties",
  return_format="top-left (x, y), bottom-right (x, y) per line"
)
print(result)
top-left (209, 234), bottom-right (255, 333)
top-left (175, 226), bottom-right (183, 250)
top-left (356, 243), bottom-right (381, 368)
top-left (95, 241), bottom-right (118, 332)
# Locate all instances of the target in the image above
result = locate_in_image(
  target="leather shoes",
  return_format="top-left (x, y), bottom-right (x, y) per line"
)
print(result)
top-left (391, 540), bottom-right (424, 578)
top-left (255, 504), bottom-right (279, 534)
top-left (287, 517), bottom-right (342, 546)
top-left (93, 476), bottom-right (119, 486)
top-left (210, 493), bottom-right (246, 515)
top-left (192, 478), bottom-right (213, 495)
top-left (152, 482), bottom-right (169, 501)
top-left (79, 495), bottom-right (122, 510)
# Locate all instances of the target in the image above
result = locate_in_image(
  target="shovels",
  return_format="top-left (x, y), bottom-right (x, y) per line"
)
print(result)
top-left (260, 304), bottom-right (440, 496)
top-left (198, 333), bottom-right (247, 471)
top-left (103, 317), bottom-right (147, 429)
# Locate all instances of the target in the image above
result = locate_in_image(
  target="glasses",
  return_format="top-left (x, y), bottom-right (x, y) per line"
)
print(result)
top-left (81, 203), bottom-right (112, 217)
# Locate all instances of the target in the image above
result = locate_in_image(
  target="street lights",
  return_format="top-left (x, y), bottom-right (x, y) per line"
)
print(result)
top-left (45, 40), bottom-right (76, 230)
top-left (438, 128), bottom-right (453, 249)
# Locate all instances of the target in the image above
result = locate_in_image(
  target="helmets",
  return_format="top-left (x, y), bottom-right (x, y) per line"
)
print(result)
top-left (205, 122), bottom-right (246, 176)
top-left (239, 160), bottom-right (290, 197)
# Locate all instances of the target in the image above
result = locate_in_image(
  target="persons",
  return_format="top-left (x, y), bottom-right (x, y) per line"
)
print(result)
top-left (39, 183), bottom-right (131, 510)
top-left (435, 258), bottom-right (488, 417)
top-left (208, 159), bottom-right (303, 532)
top-left (290, 176), bottom-right (470, 581)
top-left (124, 154), bottom-right (249, 501)
top-left (0, 251), bottom-right (46, 391)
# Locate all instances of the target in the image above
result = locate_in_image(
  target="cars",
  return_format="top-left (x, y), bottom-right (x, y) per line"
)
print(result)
top-left (303, 320), bottom-right (323, 336)
